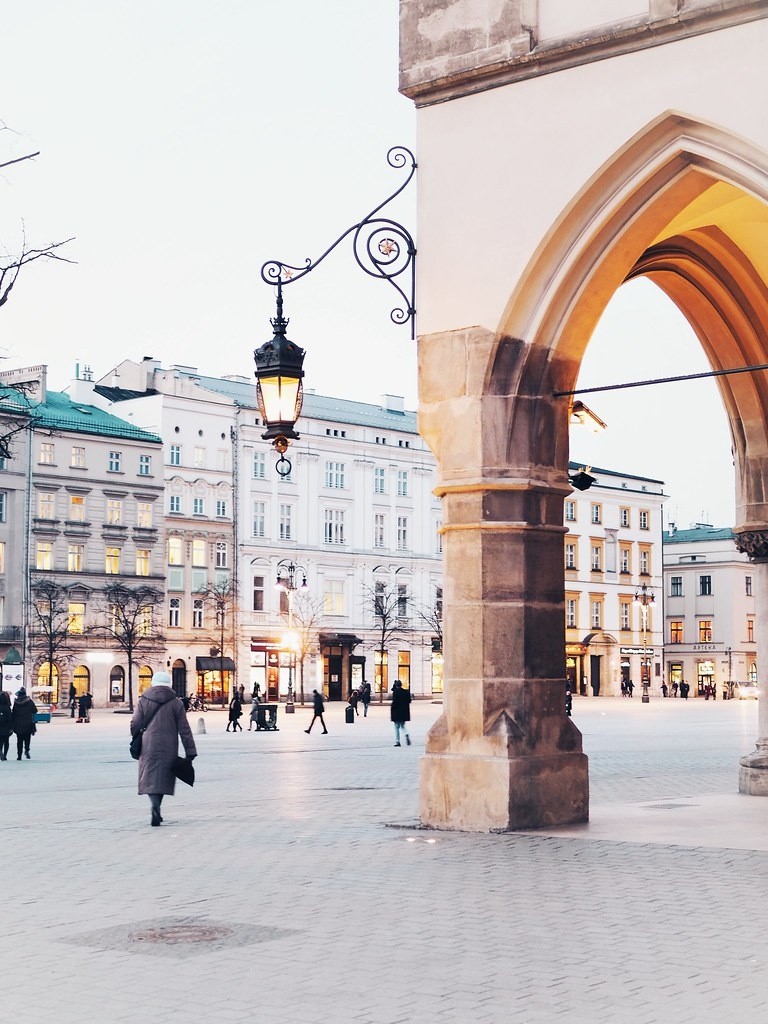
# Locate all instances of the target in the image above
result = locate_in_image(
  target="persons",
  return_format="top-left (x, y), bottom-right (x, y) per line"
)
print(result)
top-left (680, 680), bottom-right (689, 697)
top-left (76, 690), bottom-right (92, 722)
top-left (621, 679), bottom-right (635, 698)
top-left (129, 672), bottom-right (197, 826)
top-left (390, 680), bottom-right (412, 746)
top-left (0, 691), bottom-right (12, 760)
top-left (672, 683), bottom-right (679, 697)
top-left (68, 683), bottom-right (77, 708)
top-left (226, 682), bottom-right (260, 732)
top-left (705, 683), bottom-right (716, 700)
top-left (348, 680), bottom-right (371, 717)
top-left (304, 690), bottom-right (328, 734)
top-left (566, 680), bottom-right (571, 695)
top-left (11, 687), bottom-right (37, 760)
top-left (723, 681), bottom-right (729, 700)
top-left (661, 682), bottom-right (667, 697)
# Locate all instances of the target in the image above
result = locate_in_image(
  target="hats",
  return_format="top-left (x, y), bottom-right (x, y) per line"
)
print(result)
top-left (19, 688), bottom-right (26, 697)
top-left (151, 672), bottom-right (170, 687)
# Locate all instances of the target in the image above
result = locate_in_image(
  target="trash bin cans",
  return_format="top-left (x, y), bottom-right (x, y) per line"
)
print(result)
top-left (345, 706), bottom-right (354, 723)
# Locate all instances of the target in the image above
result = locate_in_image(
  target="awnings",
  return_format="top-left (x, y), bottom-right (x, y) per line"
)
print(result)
top-left (196, 657), bottom-right (234, 670)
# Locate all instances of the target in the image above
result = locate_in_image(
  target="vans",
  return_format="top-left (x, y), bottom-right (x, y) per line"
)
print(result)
top-left (721, 680), bottom-right (758, 700)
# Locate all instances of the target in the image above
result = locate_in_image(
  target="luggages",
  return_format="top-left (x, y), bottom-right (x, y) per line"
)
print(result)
top-left (345, 706), bottom-right (355, 723)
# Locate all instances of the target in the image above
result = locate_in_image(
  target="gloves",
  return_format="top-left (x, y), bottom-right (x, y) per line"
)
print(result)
top-left (185, 754), bottom-right (197, 760)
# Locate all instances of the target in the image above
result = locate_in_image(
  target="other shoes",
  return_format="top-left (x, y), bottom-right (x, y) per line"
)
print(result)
top-left (151, 808), bottom-right (163, 826)
top-left (25, 751), bottom-right (30, 759)
top-left (0, 751), bottom-right (7, 761)
top-left (406, 734), bottom-right (410, 744)
top-left (304, 730), bottom-right (310, 734)
top-left (17, 753), bottom-right (22, 760)
top-left (322, 730), bottom-right (328, 734)
top-left (394, 743), bottom-right (401, 746)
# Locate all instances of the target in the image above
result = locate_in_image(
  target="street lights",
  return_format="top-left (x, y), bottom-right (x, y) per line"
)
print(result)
top-left (632, 582), bottom-right (657, 703)
top-left (284, 631), bottom-right (300, 713)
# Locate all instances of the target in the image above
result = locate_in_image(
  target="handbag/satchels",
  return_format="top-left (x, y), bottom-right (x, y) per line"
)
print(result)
top-left (130, 730), bottom-right (142, 760)
top-left (348, 698), bottom-right (352, 703)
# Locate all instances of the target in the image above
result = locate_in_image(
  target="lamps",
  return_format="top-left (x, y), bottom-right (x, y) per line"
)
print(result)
top-left (254, 275), bottom-right (305, 476)
top-left (568, 471), bottom-right (596, 491)
